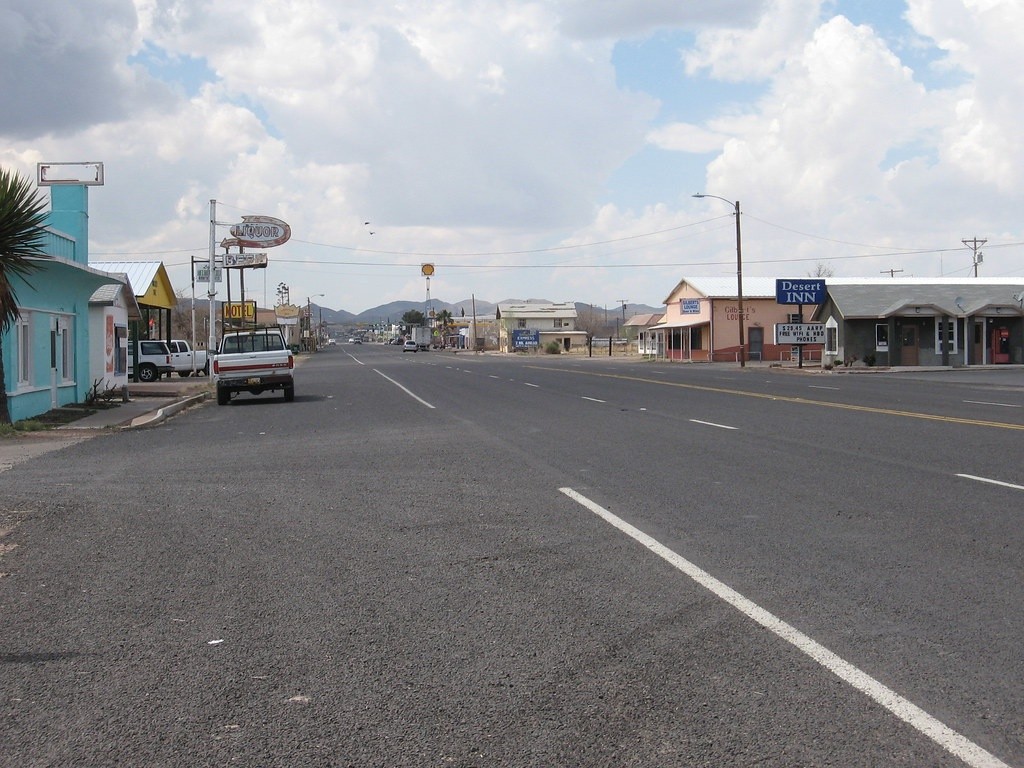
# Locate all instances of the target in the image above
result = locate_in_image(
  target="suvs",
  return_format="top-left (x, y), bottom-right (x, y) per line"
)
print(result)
top-left (213, 324), bottom-right (296, 405)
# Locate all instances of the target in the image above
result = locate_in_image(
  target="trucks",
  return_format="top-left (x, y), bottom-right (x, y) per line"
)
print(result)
top-left (411, 327), bottom-right (431, 351)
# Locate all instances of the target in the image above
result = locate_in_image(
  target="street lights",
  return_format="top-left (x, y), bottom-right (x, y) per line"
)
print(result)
top-left (308, 294), bottom-right (324, 354)
top-left (692, 194), bottom-right (745, 367)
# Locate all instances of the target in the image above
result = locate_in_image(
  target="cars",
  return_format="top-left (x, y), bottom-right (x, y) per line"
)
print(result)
top-left (354, 337), bottom-right (362, 344)
top-left (349, 338), bottom-right (354, 343)
top-left (391, 339), bottom-right (403, 345)
top-left (329, 339), bottom-right (336, 345)
top-left (403, 340), bottom-right (418, 353)
top-left (128, 341), bottom-right (175, 382)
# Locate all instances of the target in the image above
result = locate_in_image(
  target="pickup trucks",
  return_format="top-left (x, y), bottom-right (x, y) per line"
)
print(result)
top-left (161, 340), bottom-right (209, 377)
